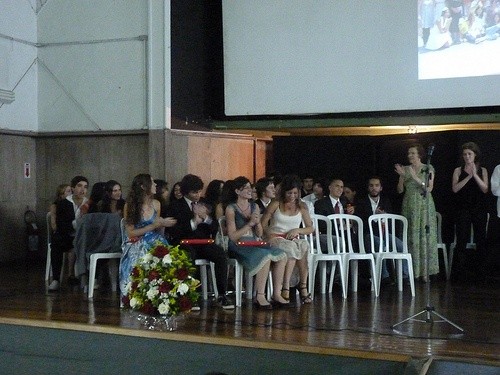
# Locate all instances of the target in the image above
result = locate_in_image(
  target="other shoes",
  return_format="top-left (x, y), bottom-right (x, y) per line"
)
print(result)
top-left (94, 282), bottom-right (102, 289)
top-left (47, 281), bottom-right (58, 291)
top-left (422, 276), bottom-right (430, 283)
top-left (452, 271), bottom-right (468, 284)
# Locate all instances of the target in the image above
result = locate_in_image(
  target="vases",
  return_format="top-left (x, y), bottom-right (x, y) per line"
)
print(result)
top-left (137, 314), bottom-right (184, 331)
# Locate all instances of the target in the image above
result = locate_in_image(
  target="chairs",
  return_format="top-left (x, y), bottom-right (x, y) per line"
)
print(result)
top-left (368, 214), bottom-right (415, 296)
top-left (297, 215), bottom-right (346, 300)
top-left (219, 215), bottom-right (273, 307)
top-left (74, 214), bottom-right (122, 298)
top-left (326, 214), bottom-right (378, 298)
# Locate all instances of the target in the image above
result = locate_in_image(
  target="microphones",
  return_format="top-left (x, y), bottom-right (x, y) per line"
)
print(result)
top-left (428, 143), bottom-right (435, 156)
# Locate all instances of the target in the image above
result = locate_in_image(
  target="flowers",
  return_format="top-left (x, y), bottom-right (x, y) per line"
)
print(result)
top-left (119, 239), bottom-right (201, 318)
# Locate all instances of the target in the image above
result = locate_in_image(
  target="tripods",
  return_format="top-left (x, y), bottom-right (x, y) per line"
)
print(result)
top-left (390, 154), bottom-right (466, 333)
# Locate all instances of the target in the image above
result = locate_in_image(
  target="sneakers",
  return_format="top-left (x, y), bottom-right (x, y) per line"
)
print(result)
top-left (217, 294), bottom-right (236, 309)
top-left (190, 301), bottom-right (200, 310)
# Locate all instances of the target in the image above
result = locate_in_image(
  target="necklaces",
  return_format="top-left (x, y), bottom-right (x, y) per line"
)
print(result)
top-left (236, 201), bottom-right (250, 212)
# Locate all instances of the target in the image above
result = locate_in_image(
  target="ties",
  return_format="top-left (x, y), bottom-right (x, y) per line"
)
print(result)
top-left (191, 203), bottom-right (195, 214)
top-left (335, 201), bottom-right (340, 224)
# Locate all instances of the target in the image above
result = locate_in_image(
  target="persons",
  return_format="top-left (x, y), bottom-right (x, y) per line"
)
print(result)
top-left (47, 173), bottom-right (410, 310)
top-left (490, 164), bottom-right (500, 218)
top-left (395, 144), bottom-right (440, 284)
top-left (451, 142), bottom-right (489, 284)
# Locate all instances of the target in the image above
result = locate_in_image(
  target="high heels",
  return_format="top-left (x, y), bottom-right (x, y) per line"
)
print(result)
top-left (252, 293), bottom-right (272, 310)
top-left (271, 296), bottom-right (296, 308)
top-left (280, 286), bottom-right (291, 305)
top-left (296, 282), bottom-right (312, 305)
